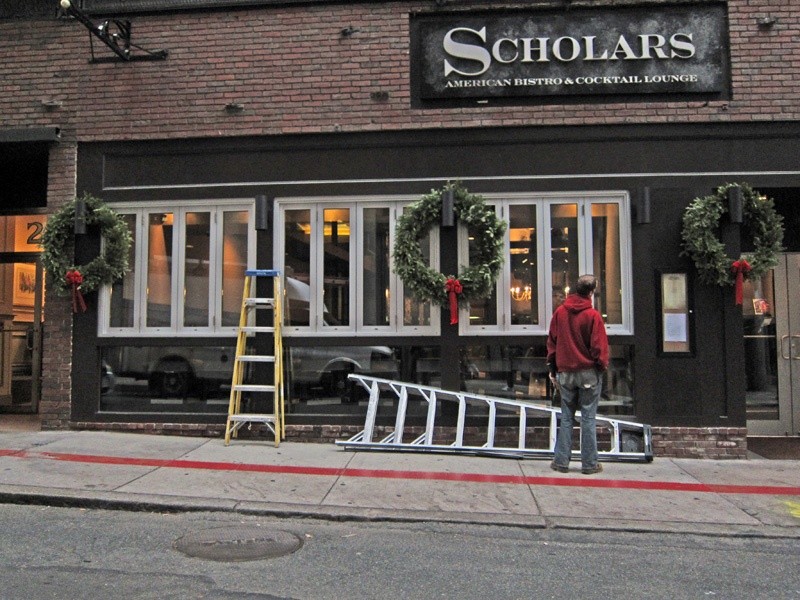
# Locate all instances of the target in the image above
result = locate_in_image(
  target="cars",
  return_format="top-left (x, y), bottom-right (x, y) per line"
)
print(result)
top-left (12, 330), bottom-right (115, 395)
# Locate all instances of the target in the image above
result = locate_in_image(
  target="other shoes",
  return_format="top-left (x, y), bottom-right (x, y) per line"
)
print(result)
top-left (581, 463), bottom-right (602, 474)
top-left (501, 385), bottom-right (515, 393)
top-left (550, 462), bottom-right (569, 473)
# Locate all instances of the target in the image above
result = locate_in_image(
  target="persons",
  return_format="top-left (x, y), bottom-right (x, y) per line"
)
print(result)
top-left (502, 347), bottom-right (514, 391)
top-left (546, 274), bottom-right (609, 474)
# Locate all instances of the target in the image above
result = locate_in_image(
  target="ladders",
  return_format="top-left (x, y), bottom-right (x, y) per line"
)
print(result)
top-left (335, 374), bottom-right (655, 464)
top-left (225, 270), bottom-right (286, 447)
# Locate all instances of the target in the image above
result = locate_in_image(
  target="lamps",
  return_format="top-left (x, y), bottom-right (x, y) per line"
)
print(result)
top-left (729, 186), bottom-right (743, 223)
top-left (73, 201), bottom-right (87, 234)
top-left (441, 190), bottom-right (455, 227)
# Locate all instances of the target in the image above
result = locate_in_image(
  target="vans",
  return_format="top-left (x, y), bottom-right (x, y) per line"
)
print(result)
top-left (102, 261), bottom-right (394, 399)
